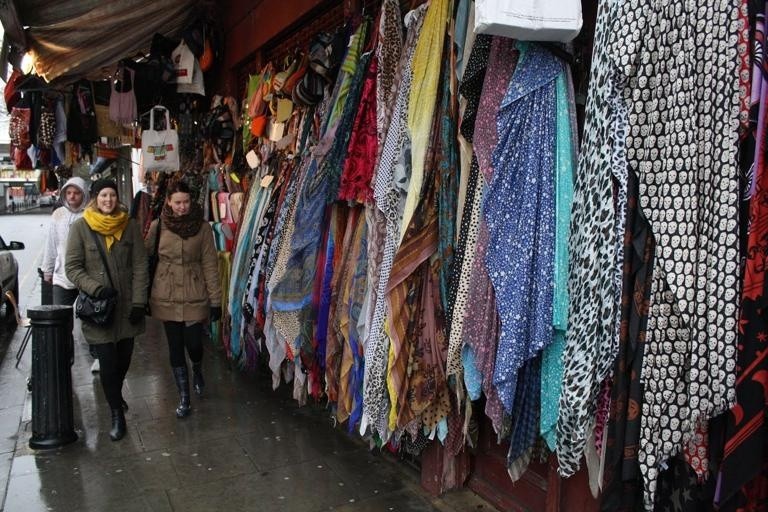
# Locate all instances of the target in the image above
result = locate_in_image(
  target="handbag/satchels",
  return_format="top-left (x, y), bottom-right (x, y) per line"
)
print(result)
top-left (35, 169), bottom-right (58, 194)
top-left (148, 255), bottom-right (159, 298)
top-left (76, 291), bottom-right (115, 326)
top-left (94, 16), bottom-right (213, 138)
top-left (142, 129), bottom-right (180, 174)
top-left (5, 67), bottom-right (98, 177)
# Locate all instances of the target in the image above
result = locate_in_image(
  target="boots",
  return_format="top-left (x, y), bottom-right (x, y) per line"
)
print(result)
top-left (109, 400), bottom-right (128, 440)
top-left (171, 366), bottom-right (191, 417)
top-left (188, 358), bottom-right (204, 395)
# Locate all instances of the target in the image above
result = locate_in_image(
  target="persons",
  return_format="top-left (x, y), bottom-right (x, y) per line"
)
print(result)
top-left (39, 176), bottom-right (101, 375)
top-left (143, 181), bottom-right (223, 418)
top-left (64, 178), bottom-right (150, 442)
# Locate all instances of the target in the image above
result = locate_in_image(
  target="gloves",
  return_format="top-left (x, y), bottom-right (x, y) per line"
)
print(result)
top-left (99, 287), bottom-right (118, 299)
top-left (144, 298), bottom-right (151, 317)
top-left (208, 303), bottom-right (222, 322)
top-left (128, 307), bottom-right (145, 325)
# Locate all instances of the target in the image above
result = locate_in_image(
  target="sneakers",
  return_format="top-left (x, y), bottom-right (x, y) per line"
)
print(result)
top-left (90, 359), bottom-right (100, 372)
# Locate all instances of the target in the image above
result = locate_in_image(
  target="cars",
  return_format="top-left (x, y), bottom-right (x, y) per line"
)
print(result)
top-left (40, 196), bottom-right (56, 207)
top-left (0, 236), bottom-right (24, 327)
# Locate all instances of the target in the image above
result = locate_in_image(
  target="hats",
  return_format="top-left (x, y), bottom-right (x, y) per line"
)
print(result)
top-left (91, 179), bottom-right (118, 197)
